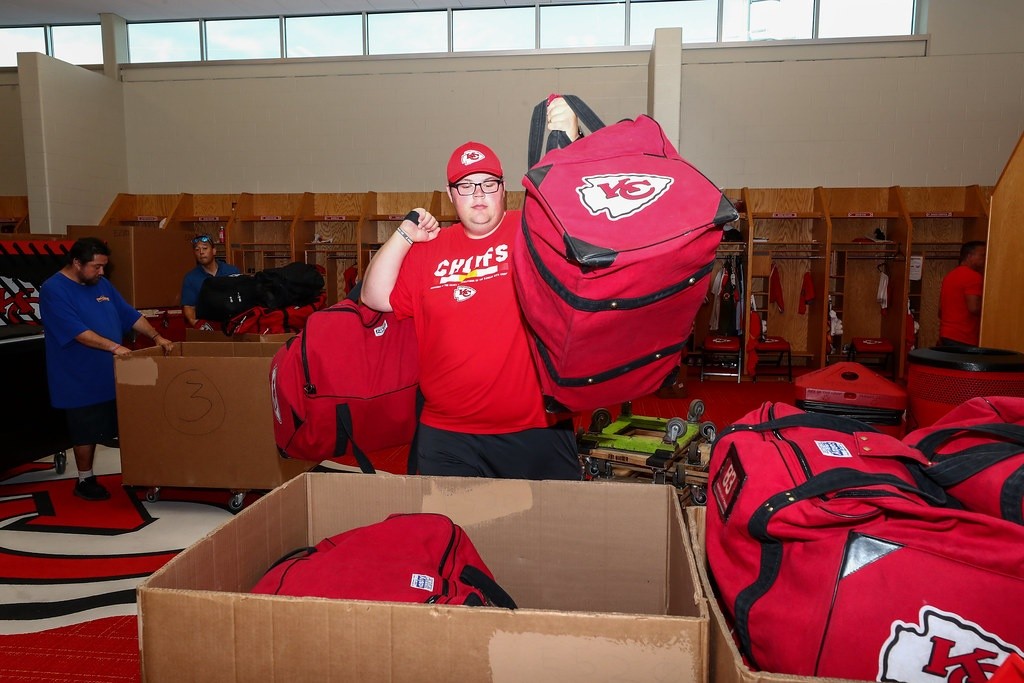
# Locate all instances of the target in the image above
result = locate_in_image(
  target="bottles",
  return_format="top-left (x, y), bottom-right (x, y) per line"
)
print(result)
top-left (219, 226), bottom-right (225, 243)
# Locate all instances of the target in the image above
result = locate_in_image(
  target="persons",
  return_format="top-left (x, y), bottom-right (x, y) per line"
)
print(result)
top-left (937, 239), bottom-right (987, 348)
top-left (182, 235), bottom-right (249, 333)
top-left (362, 93), bottom-right (588, 483)
top-left (41, 237), bottom-right (177, 503)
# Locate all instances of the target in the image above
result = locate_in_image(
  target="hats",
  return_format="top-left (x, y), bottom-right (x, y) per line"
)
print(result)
top-left (447, 141), bottom-right (503, 184)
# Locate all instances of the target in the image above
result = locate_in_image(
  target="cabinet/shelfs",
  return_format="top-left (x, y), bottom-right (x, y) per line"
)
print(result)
top-left (101, 184), bottom-right (991, 367)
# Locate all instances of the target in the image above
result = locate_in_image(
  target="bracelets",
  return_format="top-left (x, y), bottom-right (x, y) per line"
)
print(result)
top-left (111, 344), bottom-right (121, 353)
top-left (151, 332), bottom-right (162, 340)
top-left (396, 227), bottom-right (415, 245)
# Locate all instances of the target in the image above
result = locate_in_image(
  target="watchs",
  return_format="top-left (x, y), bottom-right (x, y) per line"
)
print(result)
top-left (570, 125), bottom-right (585, 142)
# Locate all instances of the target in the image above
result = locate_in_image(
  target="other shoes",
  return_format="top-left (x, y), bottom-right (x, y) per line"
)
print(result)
top-left (72, 475), bottom-right (110, 501)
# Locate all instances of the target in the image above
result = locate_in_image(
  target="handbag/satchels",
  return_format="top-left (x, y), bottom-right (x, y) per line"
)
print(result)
top-left (269, 211), bottom-right (422, 462)
top-left (249, 262), bottom-right (325, 309)
top-left (229, 290), bottom-right (329, 335)
top-left (900, 393), bottom-right (1024, 526)
top-left (251, 512), bottom-right (517, 612)
top-left (700, 400), bottom-right (1024, 683)
top-left (508, 93), bottom-right (741, 432)
top-left (195, 274), bottom-right (269, 337)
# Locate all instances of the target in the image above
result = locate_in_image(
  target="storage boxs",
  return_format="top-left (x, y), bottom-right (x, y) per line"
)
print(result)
top-left (66, 225), bottom-right (197, 308)
top-left (136, 472), bottom-right (712, 683)
top-left (684, 506), bottom-right (872, 683)
top-left (113, 324), bottom-right (322, 478)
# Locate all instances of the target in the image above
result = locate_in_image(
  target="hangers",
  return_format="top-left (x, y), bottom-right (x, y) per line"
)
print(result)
top-left (878, 255), bottom-right (891, 278)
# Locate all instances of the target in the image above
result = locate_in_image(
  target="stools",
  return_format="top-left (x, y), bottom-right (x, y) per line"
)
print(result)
top-left (848, 337), bottom-right (896, 381)
top-left (752, 336), bottom-right (792, 383)
top-left (700, 336), bottom-right (741, 383)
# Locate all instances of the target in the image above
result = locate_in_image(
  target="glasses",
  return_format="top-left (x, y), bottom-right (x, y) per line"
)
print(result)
top-left (449, 179), bottom-right (504, 195)
top-left (192, 236), bottom-right (214, 248)
